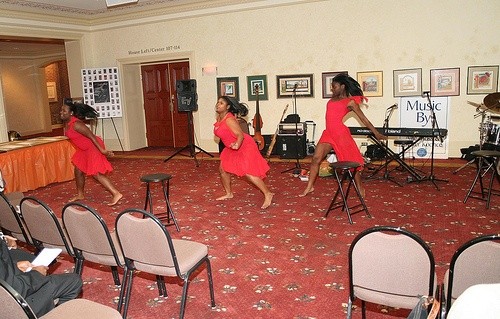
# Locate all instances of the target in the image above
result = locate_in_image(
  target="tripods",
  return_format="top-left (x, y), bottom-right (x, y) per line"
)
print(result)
top-left (367, 108), bottom-right (404, 189)
top-left (164, 111), bottom-right (214, 167)
top-left (281, 92), bottom-right (310, 175)
top-left (407, 94), bottom-right (449, 190)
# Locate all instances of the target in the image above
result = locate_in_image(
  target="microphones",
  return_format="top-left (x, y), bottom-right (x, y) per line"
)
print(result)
top-left (293, 84), bottom-right (298, 92)
top-left (387, 104), bottom-right (398, 110)
top-left (423, 91), bottom-right (430, 94)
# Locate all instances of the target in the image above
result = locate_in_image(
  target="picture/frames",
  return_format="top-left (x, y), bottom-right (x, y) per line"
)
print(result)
top-left (216, 77), bottom-right (240, 101)
top-left (467, 65), bottom-right (499, 94)
top-left (356, 70), bottom-right (383, 97)
top-left (277, 74), bottom-right (314, 98)
top-left (430, 68), bottom-right (460, 97)
top-left (322, 71), bottom-right (349, 98)
top-left (247, 75), bottom-right (268, 101)
top-left (393, 68), bottom-right (422, 97)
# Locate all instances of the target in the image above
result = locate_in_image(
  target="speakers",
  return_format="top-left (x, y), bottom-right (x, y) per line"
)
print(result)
top-left (176, 79), bottom-right (198, 112)
top-left (279, 134), bottom-right (307, 159)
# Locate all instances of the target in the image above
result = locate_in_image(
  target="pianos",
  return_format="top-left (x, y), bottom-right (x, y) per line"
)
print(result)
top-left (346, 127), bottom-right (448, 191)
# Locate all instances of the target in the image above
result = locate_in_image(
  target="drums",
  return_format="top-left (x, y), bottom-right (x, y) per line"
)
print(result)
top-left (484, 122), bottom-right (500, 147)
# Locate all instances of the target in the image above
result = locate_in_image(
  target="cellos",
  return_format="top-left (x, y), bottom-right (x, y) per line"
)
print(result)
top-left (253, 84), bottom-right (265, 150)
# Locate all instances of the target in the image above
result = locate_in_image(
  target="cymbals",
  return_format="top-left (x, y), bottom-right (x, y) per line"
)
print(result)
top-left (483, 92), bottom-right (500, 114)
top-left (468, 100), bottom-right (496, 113)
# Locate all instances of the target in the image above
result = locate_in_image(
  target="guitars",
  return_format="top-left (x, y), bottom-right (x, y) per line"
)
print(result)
top-left (265, 104), bottom-right (289, 159)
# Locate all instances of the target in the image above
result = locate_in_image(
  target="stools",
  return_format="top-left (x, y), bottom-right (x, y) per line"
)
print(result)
top-left (139, 174), bottom-right (180, 231)
top-left (324, 162), bottom-right (371, 225)
top-left (463, 151), bottom-right (500, 209)
top-left (393, 139), bottom-right (413, 172)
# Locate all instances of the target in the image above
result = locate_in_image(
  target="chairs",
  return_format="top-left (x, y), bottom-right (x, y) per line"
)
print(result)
top-left (0, 197), bottom-right (215, 319)
top-left (443, 232), bottom-right (500, 318)
top-left (347, 227), bottom-right (438, 319)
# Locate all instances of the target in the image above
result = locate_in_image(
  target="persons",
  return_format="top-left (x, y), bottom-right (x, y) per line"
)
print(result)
top-left (214, 96), bottom-right (273, 209)
top-left (298, 75), bottom-right (382, 197)
top-left (0, 238), bottom-right (82, 318)
top-left (60, 102), bottom-right (123, 206)
top-left (101, 85), bottom-right (109, 102)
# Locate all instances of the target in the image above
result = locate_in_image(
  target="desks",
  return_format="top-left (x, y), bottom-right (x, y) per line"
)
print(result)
top-left (0, 132), bottom-right (105, 194)
